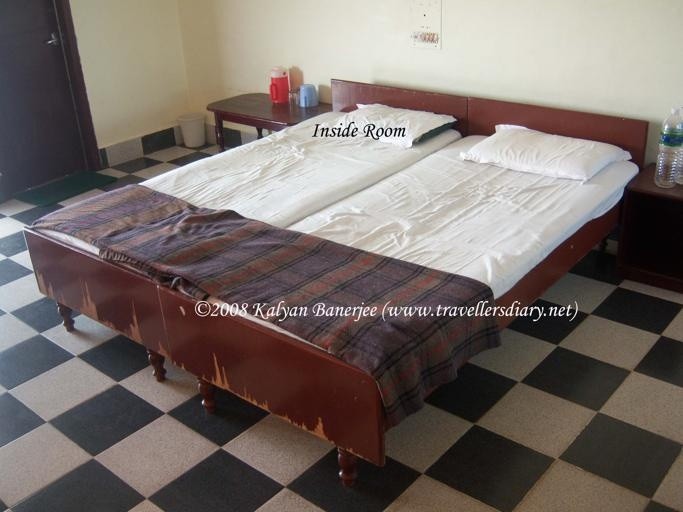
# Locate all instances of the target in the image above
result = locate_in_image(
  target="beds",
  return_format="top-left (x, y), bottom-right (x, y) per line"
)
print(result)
top-left (21, 78), bottom-right (650, 488)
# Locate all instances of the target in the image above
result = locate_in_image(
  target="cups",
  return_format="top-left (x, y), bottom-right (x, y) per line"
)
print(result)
top-left (269, 69), bottom-right (320, 107)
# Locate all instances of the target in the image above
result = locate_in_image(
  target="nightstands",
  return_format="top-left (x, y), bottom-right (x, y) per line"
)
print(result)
top-left (610, 160), bottom-right (683, 294)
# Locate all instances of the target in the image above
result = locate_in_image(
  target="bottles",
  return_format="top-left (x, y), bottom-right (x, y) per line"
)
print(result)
top-left (654, 107), bottom-right (683, 189)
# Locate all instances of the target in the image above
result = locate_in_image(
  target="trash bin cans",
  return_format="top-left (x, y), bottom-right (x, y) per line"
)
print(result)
top-left (177, 113), bottom-right (206, 148)
